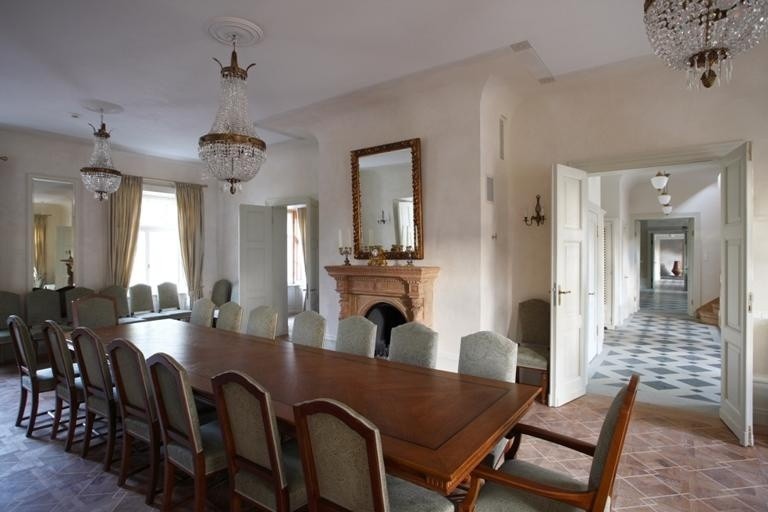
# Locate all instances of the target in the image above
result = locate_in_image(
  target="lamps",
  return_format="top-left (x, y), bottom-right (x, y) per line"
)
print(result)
top-left (641, 0), bottom-right (768, 95)
top-left (195, 15), bottom-right (268, 197)
top-left (77, 99), bottom-right (123, 203)
top-left (656, 190), bottom-right (672, 206)
top-left (649, 171), bottom-right (671, 192)
top-left (376, 209), bottom-right (391, 225)
top-left (522, 192), bottom-right (547, 228)
top-left (661, 204), bottom-right (673, 215)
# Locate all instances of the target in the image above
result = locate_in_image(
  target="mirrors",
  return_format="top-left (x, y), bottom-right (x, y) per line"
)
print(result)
top-left (25, 173), bottom-right (83, 294)
top-left (348, 136), bottom-right (427, 262)
